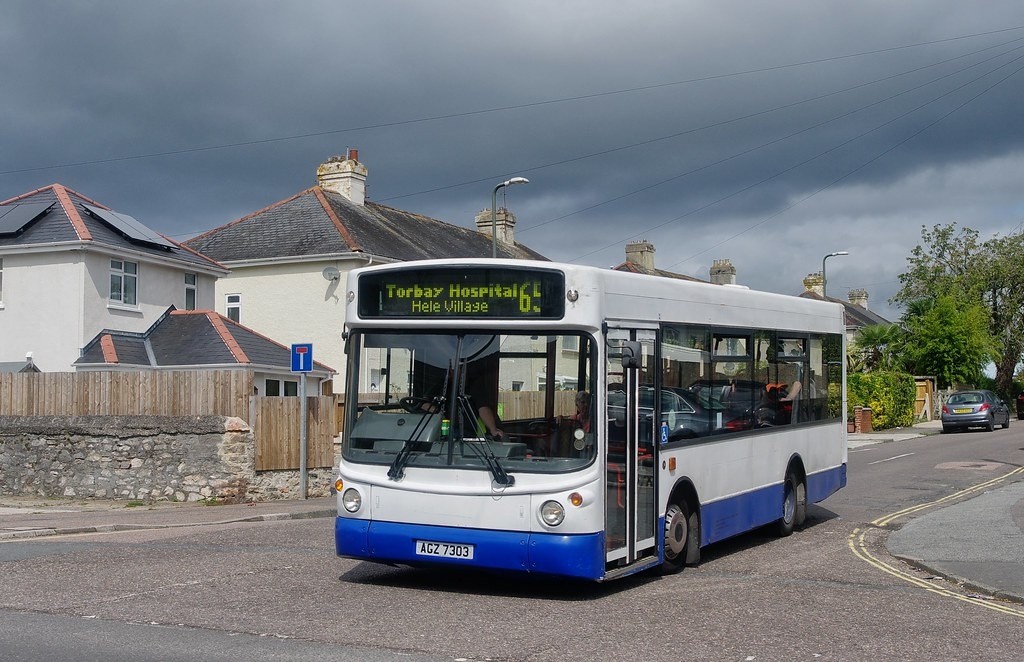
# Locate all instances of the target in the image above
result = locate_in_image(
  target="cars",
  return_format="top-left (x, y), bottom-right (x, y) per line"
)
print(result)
top-left (605, 382), bottom-right (750, 441)
top-left (685, 377), bottom-right (806, 427)
top-left (942, 389), bottom-right (1010, 434)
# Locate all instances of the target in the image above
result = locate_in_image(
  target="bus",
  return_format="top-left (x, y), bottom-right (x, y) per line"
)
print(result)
top-left (334, 258), bottom-right (849, 582)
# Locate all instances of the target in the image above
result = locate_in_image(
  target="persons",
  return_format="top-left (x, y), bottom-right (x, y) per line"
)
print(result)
top-left (756, 342), bottom-right (804, 403)
top-left (556, 390), bottom-right (591, 434)
top-left (420, 357), bottom-right (504, 439)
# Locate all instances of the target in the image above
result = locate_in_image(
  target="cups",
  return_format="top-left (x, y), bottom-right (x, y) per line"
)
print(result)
top-left (441, 420), bottom-right (450, 436)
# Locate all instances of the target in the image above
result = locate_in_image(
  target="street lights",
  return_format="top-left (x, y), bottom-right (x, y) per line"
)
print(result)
top-left (492, 176), bottom-right (531, 258)
top-left (823, 251), bottom-right (849, 302)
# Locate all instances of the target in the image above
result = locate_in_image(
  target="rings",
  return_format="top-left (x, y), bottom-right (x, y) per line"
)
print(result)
top-left (497, 429), bottom-right (500, 433)
